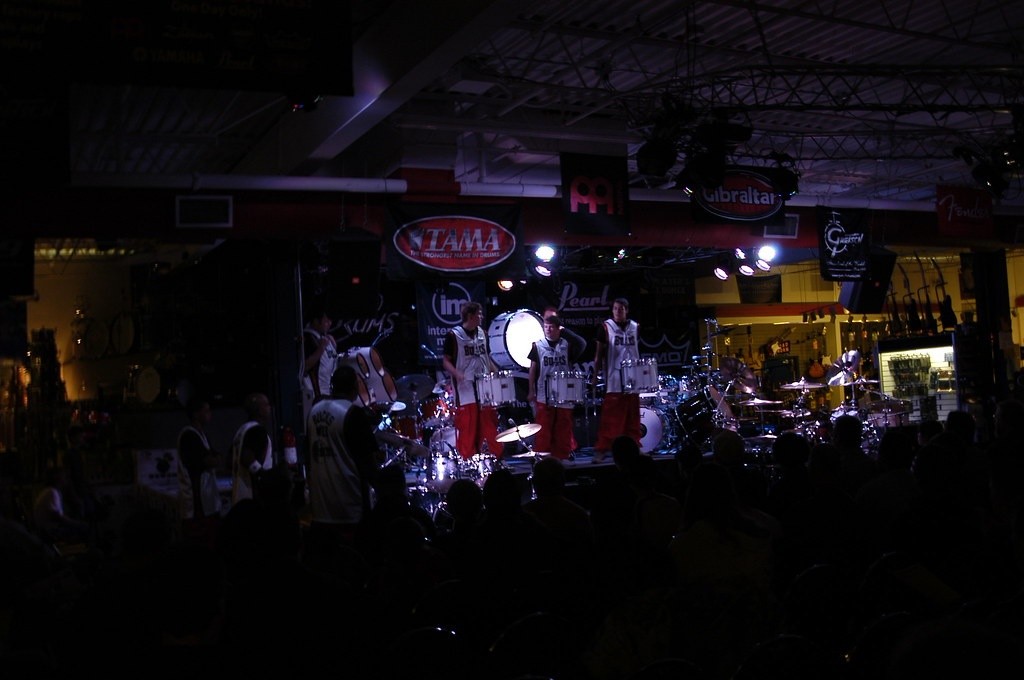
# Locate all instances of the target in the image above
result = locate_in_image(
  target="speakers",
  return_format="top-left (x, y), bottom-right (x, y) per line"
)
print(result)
top-left (839, 248), bottom-right (898, 314)
top-left (339, 263), bottom-right (393, 315)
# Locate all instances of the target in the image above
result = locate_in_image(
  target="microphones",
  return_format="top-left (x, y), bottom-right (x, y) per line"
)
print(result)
top-left (692, 355), bottom-right (708, 359)
top-left (421, 344), bottom-right (438, 358)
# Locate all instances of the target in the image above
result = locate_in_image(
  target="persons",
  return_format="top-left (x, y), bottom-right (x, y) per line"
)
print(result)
top-left (442, 301), bottom-right (516, 472)
top-left (304, 365), bottom-right (430, 525)
top-left (176, 396), bottom-right (236, 521)
top-left (298, 308), bottom-right (338, 437)
top-left (543, 307), bottom-right (586, 372)
top-left (232, 393), bottom-right (277, 506)
top-left (363, 410), bottom-right (998, 680)
top-left (591, 297), bottom-right (651, 465)
top-left (526, 316), bottom-right (577, 461)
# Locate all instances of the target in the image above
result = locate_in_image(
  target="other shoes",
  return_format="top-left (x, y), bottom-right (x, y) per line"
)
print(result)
top-left (568, 451), bottom-right (576, 463)
top-left (591, 453), bottom-right (606, 463)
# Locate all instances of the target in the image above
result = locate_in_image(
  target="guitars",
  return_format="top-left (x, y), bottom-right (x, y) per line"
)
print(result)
top-left (822, 326), bottom-right (832, 369)
top-left (887, 248), bottom-right (959, 336)
top-left (805, 328), bottom-right (824, 378)
top-left (742, 322), bottom-right (795, 373)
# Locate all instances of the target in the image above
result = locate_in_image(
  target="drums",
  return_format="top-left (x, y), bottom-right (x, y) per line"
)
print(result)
top-left (637, 404), bottom-right (675, 454)
top-left (391, 415), bottom-right (424, 445)
top-left (777, 397), bottom-right (913, 451)
top-left (404, 426), bottom-right (500, 516)
top-left (545, 371), bottom-right (588, 408)
top-left (487, 308), bottom-right (545, 371)
top-left (655, 372), bottom-right (735, 442)
top-left (474, 370), bottom-right (516, 410)
top-left (622, 357), bottom-right (660, 394)
top-left (419, 397), bottom-right (449, 427)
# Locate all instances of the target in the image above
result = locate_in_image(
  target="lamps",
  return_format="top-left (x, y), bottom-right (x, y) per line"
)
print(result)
top-left (847, 316), bottom-right (855, 332)
top-left (714, 260), bottom-right (736, 281)
top-left (810, 311), bottom-right (817, 323)
top-left (818, 310), bottom-right (825, 319)
top-left (738, 257), bottom-right (756, 276)
top-left (535, 261), bottom-right (553, 277)
top-left (803, 312), bottom-right (809, 324)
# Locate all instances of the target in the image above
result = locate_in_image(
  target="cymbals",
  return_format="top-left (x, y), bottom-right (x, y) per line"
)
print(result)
top-left (719, 357), bottom-right (757, 393)
top-left (827, 350), bottom-right (879, 385)
top-left (743, 434), bottom-right (777, 469)
top-left (782, 382), bottom-right (827, 390)
top-left (495, 421), bottom-right (553, 460)
top-left (700, 324), bottom-right (737, 338)
top-left (394, 374), bottom-right (436, 401)
top-left (377, 401), bottom-right (406, 411)
top-left (717, 415), bottom-right (758, 424)
top-left (380, 433), bottom-right (427, 457)
top-left (731, 398), bottom-right (783, 405)
